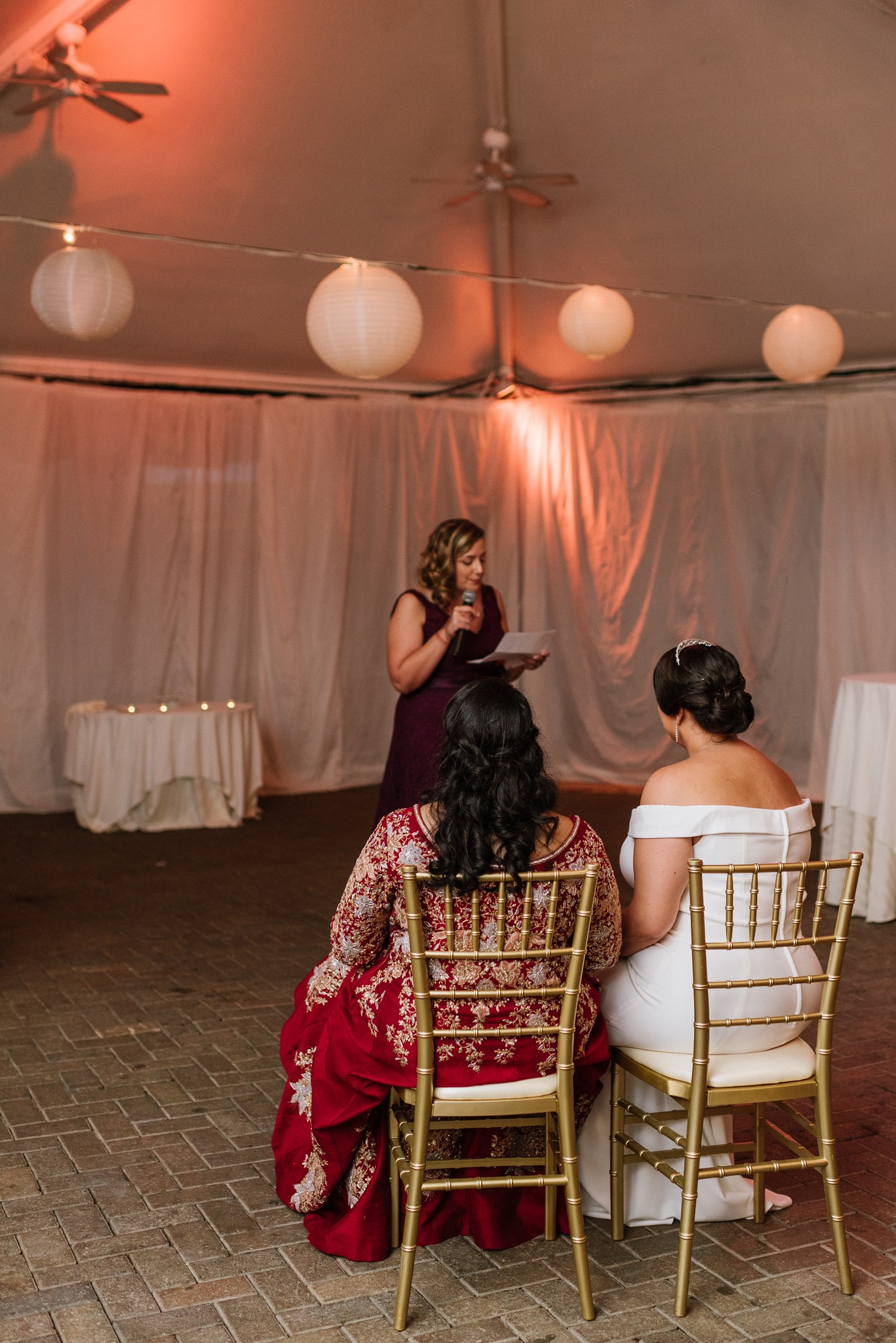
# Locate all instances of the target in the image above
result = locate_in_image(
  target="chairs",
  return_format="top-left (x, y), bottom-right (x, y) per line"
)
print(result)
top-left (607, 852), bottom-right (863, 1318)
top-left (390, 863), bottom-right (599, 1331)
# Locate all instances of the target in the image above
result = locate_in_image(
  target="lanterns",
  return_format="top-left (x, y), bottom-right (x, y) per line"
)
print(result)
top-left (31, 246), bottom-right (133, 341)
top-left (306, 263), bottom-right (423, 379)
top-left (557, 285), bottom-right (634, 360)
top-left (762, 305), bottom-right (845, 382)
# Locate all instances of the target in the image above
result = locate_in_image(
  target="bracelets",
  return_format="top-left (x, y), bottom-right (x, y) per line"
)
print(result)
top-left (435, 625), bottom-right (453, 645)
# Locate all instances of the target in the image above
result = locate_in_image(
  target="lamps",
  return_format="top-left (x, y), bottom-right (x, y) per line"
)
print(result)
top-left (761, 305), bottom-right (846, 384)
top-left (308, 263), bottom-right (421, 378)
top-left (560, 284), bottom-right (633, 357)
top-left (30, 246), bottom-right (136, 341)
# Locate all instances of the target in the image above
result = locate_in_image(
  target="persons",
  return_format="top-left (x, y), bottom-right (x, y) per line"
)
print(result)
top-left (373, 518), bottom-right (550, 827)
top-left (574, 639), bottom-right (824, 1225)
top-left (272, 677), bottom-right (622, 1260)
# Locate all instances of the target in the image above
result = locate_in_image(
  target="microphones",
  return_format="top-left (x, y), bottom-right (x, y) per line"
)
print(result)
top-left (458, 589), bottom-right (475, 653)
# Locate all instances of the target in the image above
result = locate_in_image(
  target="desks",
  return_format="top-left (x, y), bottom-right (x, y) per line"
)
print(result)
top-left (62, 701), bottom-right (263, 835)
top-left (818, 670), bottom-right (896, 922)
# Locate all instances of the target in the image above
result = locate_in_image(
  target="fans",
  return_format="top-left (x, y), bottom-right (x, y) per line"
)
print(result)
top-left (0, 22), bottom-right (169, 122)
top-left (408, 131), bottom-right (576, 209)
